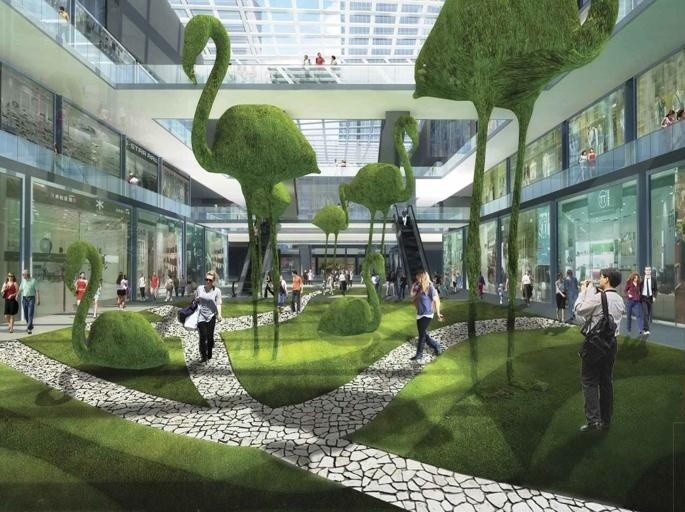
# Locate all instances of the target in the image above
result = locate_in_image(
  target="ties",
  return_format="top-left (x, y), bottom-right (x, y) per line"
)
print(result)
top-left (647, 278), bottom-right (649, 297)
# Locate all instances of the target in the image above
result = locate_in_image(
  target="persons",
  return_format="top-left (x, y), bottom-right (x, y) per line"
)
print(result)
top-left (193, 268), bottom-right (223, 363)
top-left (398, 209), bottom-right (410, 249)
top-left (579, 150), bottom-right (588, 181)
top-left (56, 6), bottom-right (69, 44)
top-left (303, 54), bottom-right (311, 78)
top-left (127, 172), bottom-right (139, 186)
top-left (253, 219), bottom-right (269, 245)
top-left (331, 54), bottom-right (338, 83)
top-left (676, 108), bottom-right (685, 119)
top-left (588, 148), bottom-right (597, 178)
top-left (315, 52), bottom-right (325, 65)
top-left (572, 268), bottom-right (626, 432)
top-left (660, 110), bottom-right (676, 151)
top-left (15, 269), bottom-right (40, 334)
top-left (340, 159), bottom-right (346, 167)
top-left (74, 270), bottom-right (193, 318)
top-left (409, 271), bottom-right (444, 360)
top-left (1, 272), bottom-right (18, 333)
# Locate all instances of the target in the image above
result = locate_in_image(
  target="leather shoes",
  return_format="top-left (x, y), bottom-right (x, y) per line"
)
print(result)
top-left (580, 422), bottom-right (610, 433)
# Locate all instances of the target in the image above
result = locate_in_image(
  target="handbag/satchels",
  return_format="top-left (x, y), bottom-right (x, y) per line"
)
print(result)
top-left (178, 299), bottom-right (200, 330)
top-left (1, 291), bottom-right (5, 297)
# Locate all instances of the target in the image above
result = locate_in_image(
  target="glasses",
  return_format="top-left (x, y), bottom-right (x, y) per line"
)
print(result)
top-left (7, 275), bottom-right (14, 278)
top-left (204, 278), bottom-right (212, 282)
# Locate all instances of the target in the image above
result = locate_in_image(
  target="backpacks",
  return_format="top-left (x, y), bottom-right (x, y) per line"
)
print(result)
top-left (579, 292), bottom-right (618, 367)
